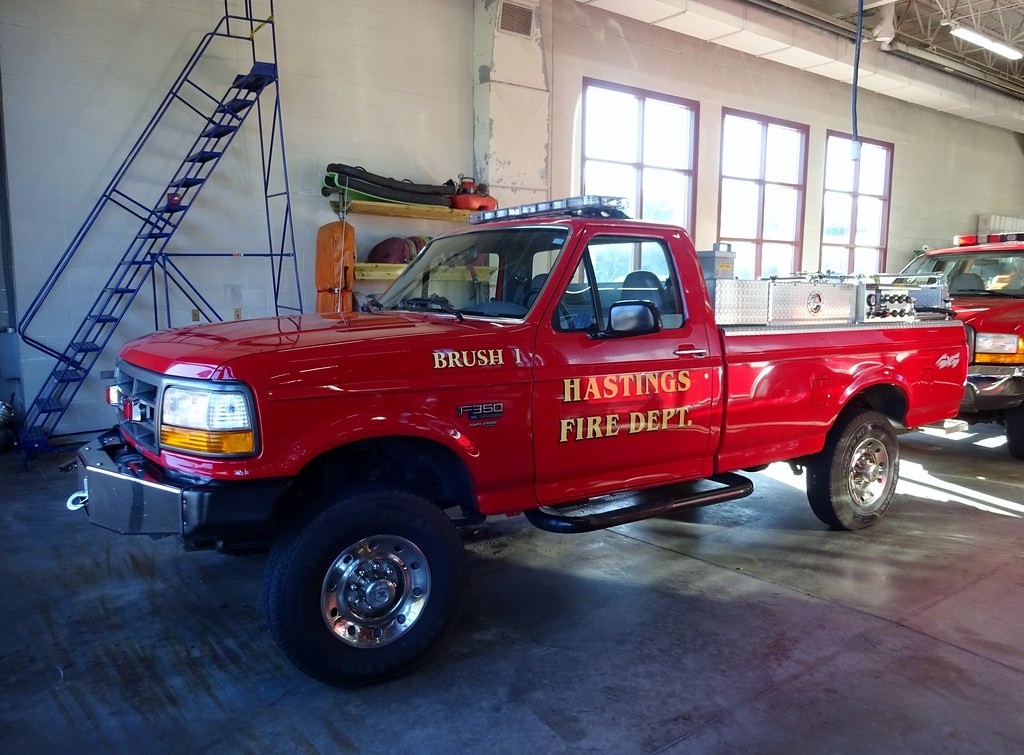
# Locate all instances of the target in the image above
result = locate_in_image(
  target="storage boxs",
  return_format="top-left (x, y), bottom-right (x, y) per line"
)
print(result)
top-left (696, 243), bottom-right (736, 278)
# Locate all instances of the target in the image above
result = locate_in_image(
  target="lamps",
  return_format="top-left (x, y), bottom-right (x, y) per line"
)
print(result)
top-left (949, 22), bottom-right (1023, 60)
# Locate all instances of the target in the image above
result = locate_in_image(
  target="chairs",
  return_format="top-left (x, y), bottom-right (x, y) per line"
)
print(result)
top-left (622, 271), bottom-right (668, 315)
top-left (526, 274), bottom-right (567, 312)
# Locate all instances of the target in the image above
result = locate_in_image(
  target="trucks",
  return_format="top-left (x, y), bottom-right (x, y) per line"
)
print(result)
top-left (55, 193), bottom-right (972, 688)
top-left (893, 232), bottom-right (1024, 462)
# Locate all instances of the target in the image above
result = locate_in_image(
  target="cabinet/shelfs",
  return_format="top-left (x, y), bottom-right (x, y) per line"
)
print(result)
top-left (330, 200), bottom-right (498, 283)
